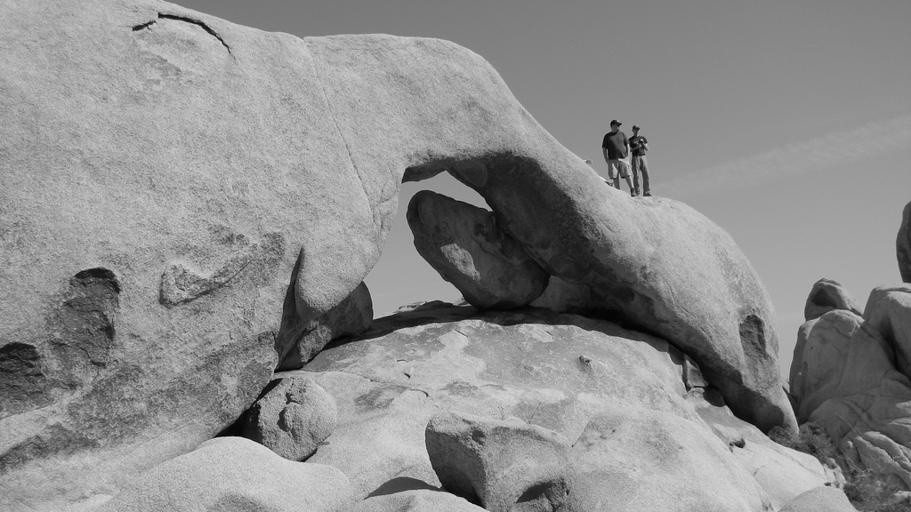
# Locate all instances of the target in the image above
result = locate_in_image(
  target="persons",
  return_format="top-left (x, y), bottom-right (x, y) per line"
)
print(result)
top-left (603, 120), bottom-right (637, 197)
top-left (629, 125), bottom-right (653, 196)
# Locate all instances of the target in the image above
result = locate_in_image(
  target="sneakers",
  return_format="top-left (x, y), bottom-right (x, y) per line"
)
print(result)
top-left (630, 188), bottom-right (651, 197)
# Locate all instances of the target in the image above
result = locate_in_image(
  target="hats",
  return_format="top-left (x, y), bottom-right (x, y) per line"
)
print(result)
top-left (632, 124), bottom-right (641, 131)
top-left (609, 120), bottom-right (622, 128)
